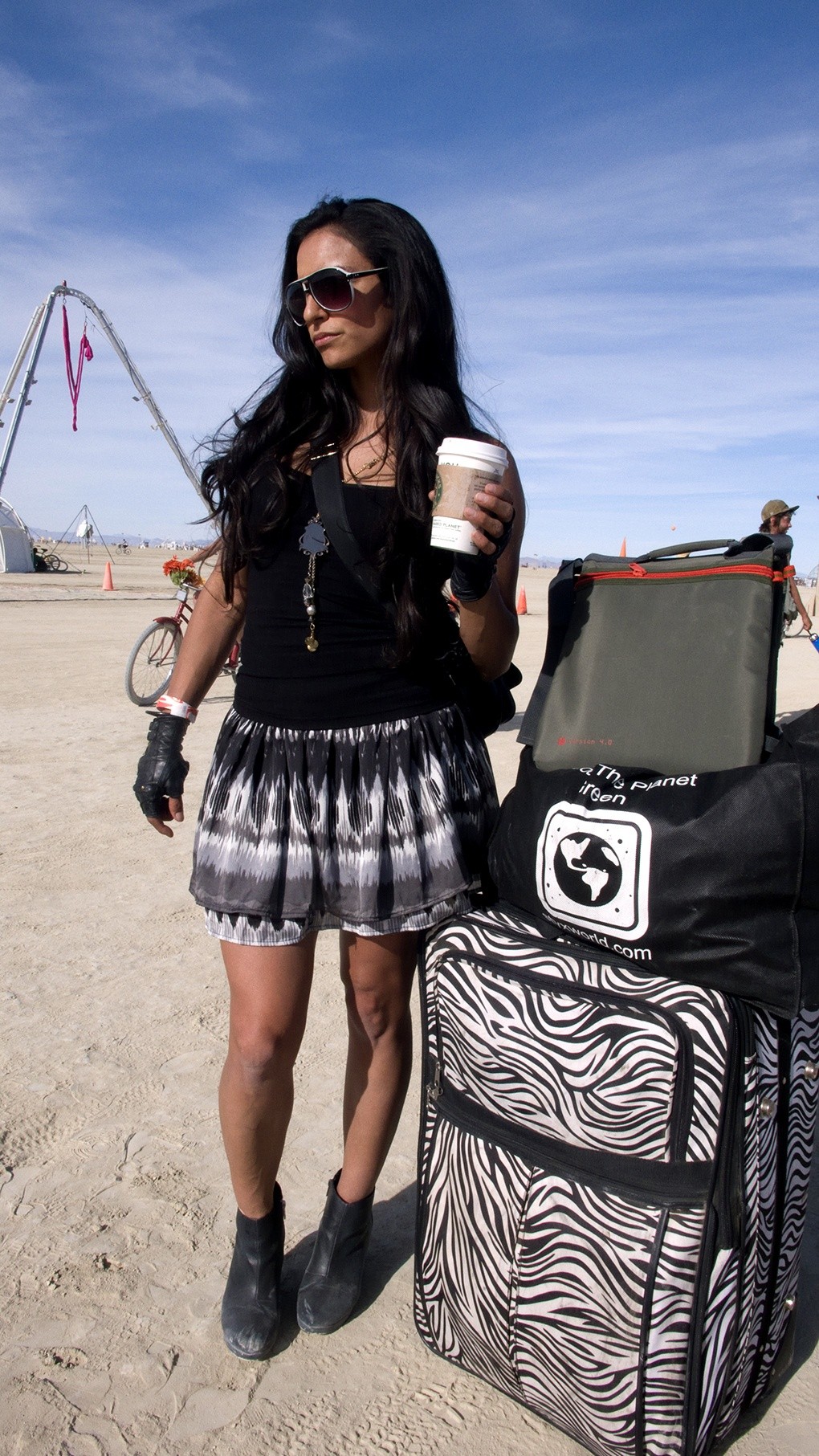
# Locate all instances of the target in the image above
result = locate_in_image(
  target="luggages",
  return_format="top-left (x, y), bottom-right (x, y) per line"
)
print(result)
top-left (413, 908), bottom-right (819, 1456)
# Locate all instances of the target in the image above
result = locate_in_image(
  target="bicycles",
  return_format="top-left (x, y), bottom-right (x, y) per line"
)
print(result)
top-left (32, 546), bottom-right (69, 571)
top-left (781, 606), bottom-right (807, 637)
top-left (125, 576), bottom-right (242, 707)
top-left (114, 542), bottom-right (132, 555)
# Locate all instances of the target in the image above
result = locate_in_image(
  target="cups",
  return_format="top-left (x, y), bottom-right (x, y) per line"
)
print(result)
top-left (430, 436), bottom-right (509, 554)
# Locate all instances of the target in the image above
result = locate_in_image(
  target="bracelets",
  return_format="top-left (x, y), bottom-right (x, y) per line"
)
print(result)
top-left (157, 694), bottom-right (199, 725)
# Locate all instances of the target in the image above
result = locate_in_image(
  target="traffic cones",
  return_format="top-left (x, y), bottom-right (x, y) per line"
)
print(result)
top-left (102, 561), bottom-right (120, 591)
top-left (517, 586), bottom-right (530, 615)
top-left (450, 592), bottom-right (460, 615)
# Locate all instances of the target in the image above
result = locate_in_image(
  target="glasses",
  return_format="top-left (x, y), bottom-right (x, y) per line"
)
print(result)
top-left (286, 267), bottom-right (391, 326)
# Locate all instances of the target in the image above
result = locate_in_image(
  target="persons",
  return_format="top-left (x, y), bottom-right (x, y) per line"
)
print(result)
top-left (134, 197), bottom-right (524, 1358)
top-left (759, 499), bottom-right (813, 639)
top-left (31, 548), bottom-right (44, 571)
top-left (182, 535), bottom-right (245, 671)
top-left (122, 539), bottom-right (128, 553)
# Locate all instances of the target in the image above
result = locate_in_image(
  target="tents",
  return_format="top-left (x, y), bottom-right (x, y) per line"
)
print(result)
top-left (0, 497), bottom-right (34, 573)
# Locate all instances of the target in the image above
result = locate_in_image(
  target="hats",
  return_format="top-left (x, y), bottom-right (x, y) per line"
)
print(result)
top-left (761, 500), bottom-right (799, 522)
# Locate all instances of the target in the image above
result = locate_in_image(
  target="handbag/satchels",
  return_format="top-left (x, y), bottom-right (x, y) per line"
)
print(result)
top-left (451, 624), bottom-right (523, 737)
top-left (480, 704), bottom-right (819, 1018)
top-left (516, 535), bottom-right (796, 777)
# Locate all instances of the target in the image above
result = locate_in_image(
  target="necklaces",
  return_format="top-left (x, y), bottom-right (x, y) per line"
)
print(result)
top-left (298, 450), bottom-right (393, 652)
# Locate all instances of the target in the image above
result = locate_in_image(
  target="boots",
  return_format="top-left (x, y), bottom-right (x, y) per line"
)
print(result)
top-left (222, 1182), bottom-right (285, 1357)
top-left (297, 1169), bottom-right (375, 1334)
